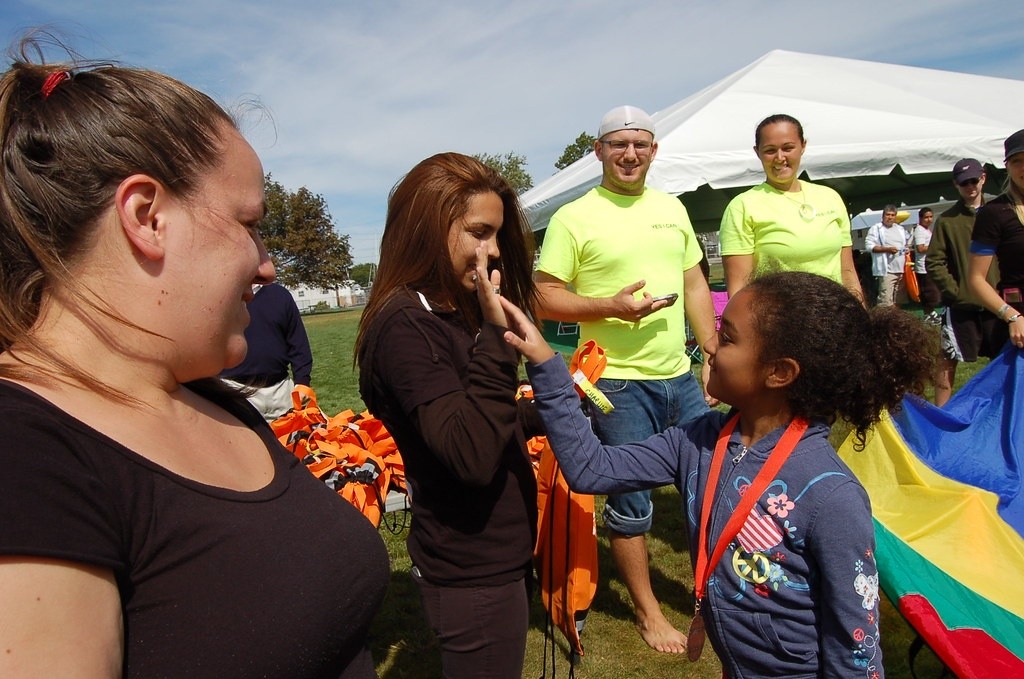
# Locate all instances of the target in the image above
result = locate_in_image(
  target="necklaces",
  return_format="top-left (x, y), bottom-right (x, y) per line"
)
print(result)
top-left (775, 187), bottom-right (816, 220)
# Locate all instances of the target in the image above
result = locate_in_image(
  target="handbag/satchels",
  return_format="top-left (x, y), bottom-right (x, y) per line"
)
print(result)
top-left (904, 252), bottom-right (921, 304)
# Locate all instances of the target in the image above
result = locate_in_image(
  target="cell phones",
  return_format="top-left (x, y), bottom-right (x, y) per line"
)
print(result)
top-left (652, 293), bottom-right (678, 307)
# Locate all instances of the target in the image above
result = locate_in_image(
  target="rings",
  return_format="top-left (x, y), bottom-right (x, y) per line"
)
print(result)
top-left (493, 288), bottom-right (501, 295)
top-left (472, 274), bottom-right (478, 281)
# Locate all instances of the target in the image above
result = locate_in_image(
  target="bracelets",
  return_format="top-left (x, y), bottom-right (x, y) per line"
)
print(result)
top-left (995, 304), bottom-right (1011, 319)
top-left (1006, 313), bottom-right (1023, 326)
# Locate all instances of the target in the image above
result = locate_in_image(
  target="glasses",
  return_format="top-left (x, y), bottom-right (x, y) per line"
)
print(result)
top-left (959, 177), bottom-right (983, 187)
top-left (602, 138), bottom-right (653, 151)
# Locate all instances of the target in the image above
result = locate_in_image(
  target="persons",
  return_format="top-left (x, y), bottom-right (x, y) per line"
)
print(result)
top-left (864, 204), bottom-right (912, 314)
top-left (0, 30), bottom-right (391, 679)
top-left (925, 159), bottom-right (1003, 410)
top-left (965, 127), bottom-right (1024, 355)
top-left (720, 113), bottom-right (869, 315)
top-left (497, 271), bottom-right (950, 679)
top-left (352, 152), bottom-right (549, 679)
top-left (214, 281), bottom-right (315, 428)
top-left (526, 104), bottom-right (722, 654)
top-left (912, 207), bottom-right (934, 323)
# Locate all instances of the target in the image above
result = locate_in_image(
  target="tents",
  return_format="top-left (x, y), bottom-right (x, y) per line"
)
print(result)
top-left (513, 49), bottom-right (1024, 232)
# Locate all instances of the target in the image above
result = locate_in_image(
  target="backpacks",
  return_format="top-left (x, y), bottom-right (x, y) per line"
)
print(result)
top-left (533, 338), bottom-right (609, 679)
top-left (271, 383), bottom-right (410, 536)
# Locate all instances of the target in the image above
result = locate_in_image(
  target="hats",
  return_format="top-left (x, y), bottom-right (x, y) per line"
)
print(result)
top-left (953, 158), bottom-right (986, 185)
top-left (598, 106), bottom-right (655, 145)
top-left (1004, 129), bottom-right (1024, 162)
